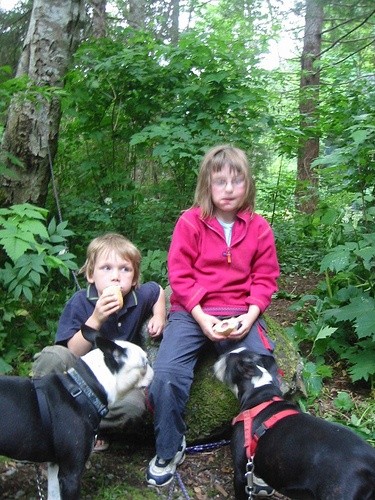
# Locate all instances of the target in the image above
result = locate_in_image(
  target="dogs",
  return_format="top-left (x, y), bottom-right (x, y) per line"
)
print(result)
top-left (0, 324), bottom-right (155, 500)
top-left (213, 346), bottom-right (375, 500)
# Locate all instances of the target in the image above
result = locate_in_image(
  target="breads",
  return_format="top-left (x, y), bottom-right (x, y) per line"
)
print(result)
top-left (213, 317), bottom-right (239, 336)
top-left (102, 285), bottom-right (124, 314)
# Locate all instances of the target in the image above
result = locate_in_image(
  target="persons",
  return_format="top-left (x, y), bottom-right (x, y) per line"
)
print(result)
top-left (143, 142), bottom-right (283, 491)
top-left (31, 233), bottom-right (167, 452)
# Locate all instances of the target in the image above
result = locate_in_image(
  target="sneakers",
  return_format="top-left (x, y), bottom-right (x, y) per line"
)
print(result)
top-left (144, 435), bottom-right (187, 487)
